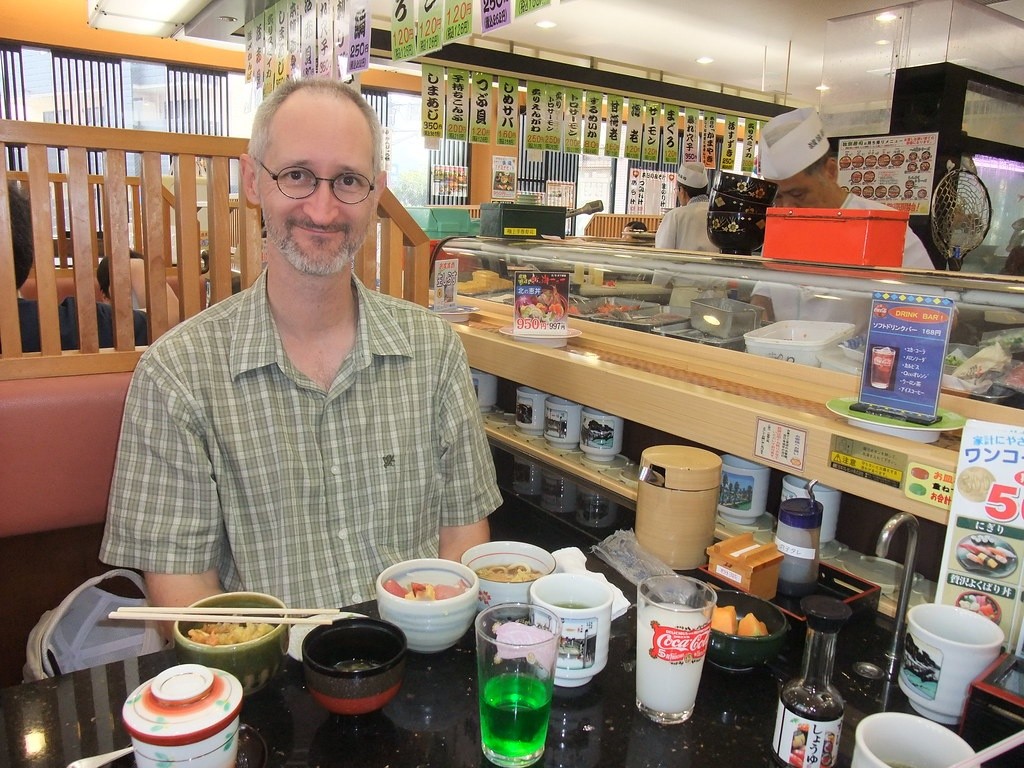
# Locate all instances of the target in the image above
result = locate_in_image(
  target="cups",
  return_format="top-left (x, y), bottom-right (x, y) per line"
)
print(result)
top-left (850, 603), bottom-right (1004, 768)
top-left (529, 573), bottom-right (614, 687)
top-left (635, 574), bottom-right (718, 726)
top-left (474, 603), bottom-right (562, 768)
top-left (871, 347), bottom-right (896, 389)
top-left (781, 475), bottom-right (842, 549)
top-left (468, 366), bottom-right (623, 527)
top-left (121, 664), bottom-right (243, 768)
top-left (718, 454), bottom-right (771, 525)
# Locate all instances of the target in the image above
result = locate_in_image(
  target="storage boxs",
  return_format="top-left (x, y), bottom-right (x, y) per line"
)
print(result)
top-left (479, 202), bottom-right (567, 240)
top-left (743, 319), bottom-right (857, 368)
top-left (761, 204), bottom-right (909, 268)
top-left (698, 546), bottom-right (880, 670)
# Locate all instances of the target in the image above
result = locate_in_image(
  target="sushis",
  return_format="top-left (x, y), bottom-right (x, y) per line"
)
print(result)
top-left (516, 290), bottom-right (566, 325)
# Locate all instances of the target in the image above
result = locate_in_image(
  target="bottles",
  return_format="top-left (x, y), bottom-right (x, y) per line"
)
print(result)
top-left (773, 479), bottom-right (824, 596)
top-left (770, 596), bottom-right (853, 768)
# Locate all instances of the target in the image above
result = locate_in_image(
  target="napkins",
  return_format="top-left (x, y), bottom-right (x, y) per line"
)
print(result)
top-left (550, 548), bottom-right (630, 621)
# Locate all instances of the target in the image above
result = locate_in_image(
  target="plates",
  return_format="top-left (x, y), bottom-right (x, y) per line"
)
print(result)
top-left (287, 611), bottom-right (369, 662)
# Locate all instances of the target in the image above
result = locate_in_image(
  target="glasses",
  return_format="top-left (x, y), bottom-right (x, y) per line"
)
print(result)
top-left (260, 163), bottom-right (374, 204)
top-left (674, 188), bottom-right (679, 193)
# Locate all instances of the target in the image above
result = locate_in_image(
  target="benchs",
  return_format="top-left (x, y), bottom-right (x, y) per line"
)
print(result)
top-left (21, 277), bottom-right (206, 326)
top-left (0, 371), bottom-right (154, 685)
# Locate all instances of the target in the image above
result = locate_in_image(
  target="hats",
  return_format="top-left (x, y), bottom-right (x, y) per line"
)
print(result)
top-left (676, 162), bottom-right (708, 188)
top-left (22, 569), bottom-right (174, 683)
top-left (761, 108), bottom-right (830, 181)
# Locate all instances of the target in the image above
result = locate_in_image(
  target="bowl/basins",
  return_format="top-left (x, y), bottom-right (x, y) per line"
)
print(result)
top-left (707, 171), bottom-right (779, 255)
top-left (706, 590), bottom-right (790, 671)
top-left (460, 539), bottom-right (557, 612)
top-left (173, 591), bottom-right (290, 697)
top-left (303, 619), bottom-right (407, 716)
top-left (375, 558), bottom-right (480, 655)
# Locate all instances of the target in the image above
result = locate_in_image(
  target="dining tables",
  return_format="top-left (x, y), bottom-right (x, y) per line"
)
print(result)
top-left (0, 540), bottom-right (1024, 768)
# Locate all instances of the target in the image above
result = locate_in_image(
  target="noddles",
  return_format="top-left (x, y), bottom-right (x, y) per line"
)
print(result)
top-left (475, 562), bottom-right (543, 582)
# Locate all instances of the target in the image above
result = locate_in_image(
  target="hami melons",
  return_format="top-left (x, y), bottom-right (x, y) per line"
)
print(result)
top-left (704, 605), bottom-right (769, 636)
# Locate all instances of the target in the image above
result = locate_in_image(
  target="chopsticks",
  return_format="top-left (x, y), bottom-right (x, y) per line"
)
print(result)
top-left (109, 606), bottom-right (340, 626)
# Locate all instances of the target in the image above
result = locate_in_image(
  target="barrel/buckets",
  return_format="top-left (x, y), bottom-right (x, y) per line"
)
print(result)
top-left (634, 445), bottom-right (722, 571)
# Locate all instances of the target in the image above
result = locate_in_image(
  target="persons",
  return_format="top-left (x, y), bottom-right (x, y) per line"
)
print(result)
top-left (748, 106), bottom-right (960, 337)
top-left (0, 182), bottom-right (179, 356)
top-left (98, 70), bottom-right (504, 643)
top-left (651, 162), bottom-right (729, 306)
top-left (618, 222), bottom-right (652, 302)
